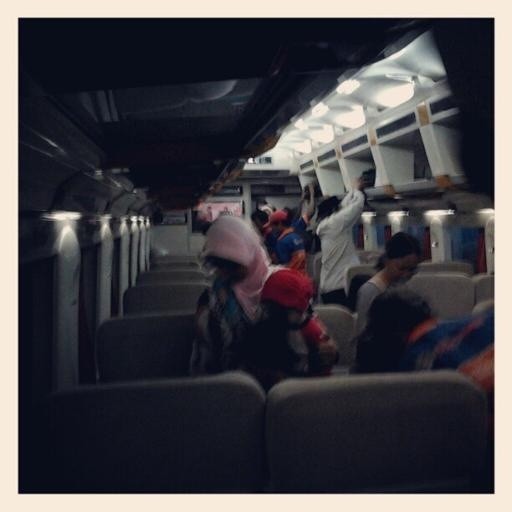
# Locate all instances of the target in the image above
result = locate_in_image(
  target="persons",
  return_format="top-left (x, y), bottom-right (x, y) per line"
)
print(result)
top-left (243, 268), bottom-right (340, 388)
top-left (187, 210), bottom-right (288, 386)
top-left (367, 287), bottom-right (494, 414)
top-left (252, 173), bottom-right (366, 308)
top-left (347, 232), bottom-right (423, 371)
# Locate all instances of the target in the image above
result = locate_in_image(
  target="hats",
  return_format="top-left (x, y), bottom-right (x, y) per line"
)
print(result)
top-left (261, 270), bottom-right (313, 311)
top-left (262, 210), bottom-right (287, 229)
